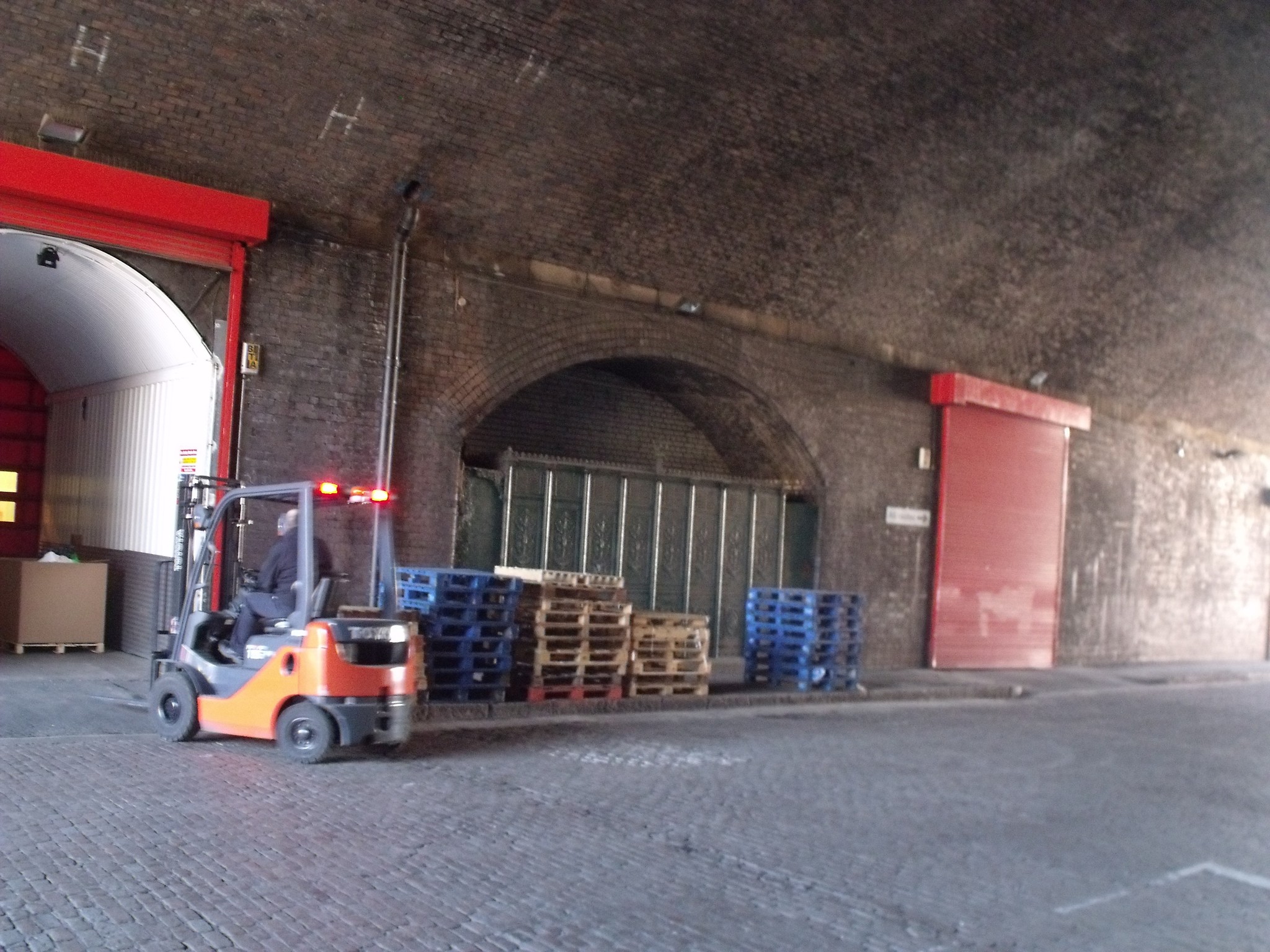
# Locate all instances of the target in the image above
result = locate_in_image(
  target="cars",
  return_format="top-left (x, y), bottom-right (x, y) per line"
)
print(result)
top-left (150, 478), bottom-right (425, 762)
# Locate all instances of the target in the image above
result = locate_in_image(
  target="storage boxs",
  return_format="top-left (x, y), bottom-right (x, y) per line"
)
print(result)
top-left (0, 558), bottom-right (109, 644)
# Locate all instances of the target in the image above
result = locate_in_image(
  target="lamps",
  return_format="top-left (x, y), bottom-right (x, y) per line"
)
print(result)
top-left (36, 113), bottom-right (88, 158)
top-left (1026, 370), bottom-right (1048, 393)
top-left (36, 243), bottom-right (60, 269)
top-left (675, 293), bottom-right (704, 316)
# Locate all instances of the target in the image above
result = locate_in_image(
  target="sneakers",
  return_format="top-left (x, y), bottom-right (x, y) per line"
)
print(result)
top-left (219, 639), bottom-right (245, 665)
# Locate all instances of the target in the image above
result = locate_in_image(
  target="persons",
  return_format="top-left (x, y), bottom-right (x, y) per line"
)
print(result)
top-left (215, 507), bottom-right (333, 664)
